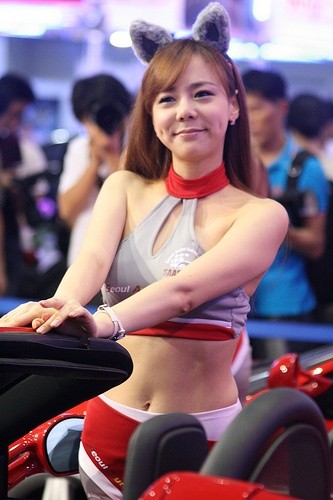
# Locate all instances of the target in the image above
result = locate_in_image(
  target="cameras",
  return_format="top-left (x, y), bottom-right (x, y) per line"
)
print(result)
top-left (90, 100), bottom-right (126, 135)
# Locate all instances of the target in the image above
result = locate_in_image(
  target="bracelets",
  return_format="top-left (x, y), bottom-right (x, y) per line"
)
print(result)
top-left (99, 304), bottom-right (125, 343)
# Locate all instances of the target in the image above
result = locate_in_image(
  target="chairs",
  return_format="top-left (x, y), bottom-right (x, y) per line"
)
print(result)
top-left (123, 413), bottom-right (208, 500)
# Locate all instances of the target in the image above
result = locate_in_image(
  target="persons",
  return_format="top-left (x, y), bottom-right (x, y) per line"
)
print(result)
top-left (0, 0), bottom-right (288, 500)
top-left (0, 70), bottom-right (333, 365)
